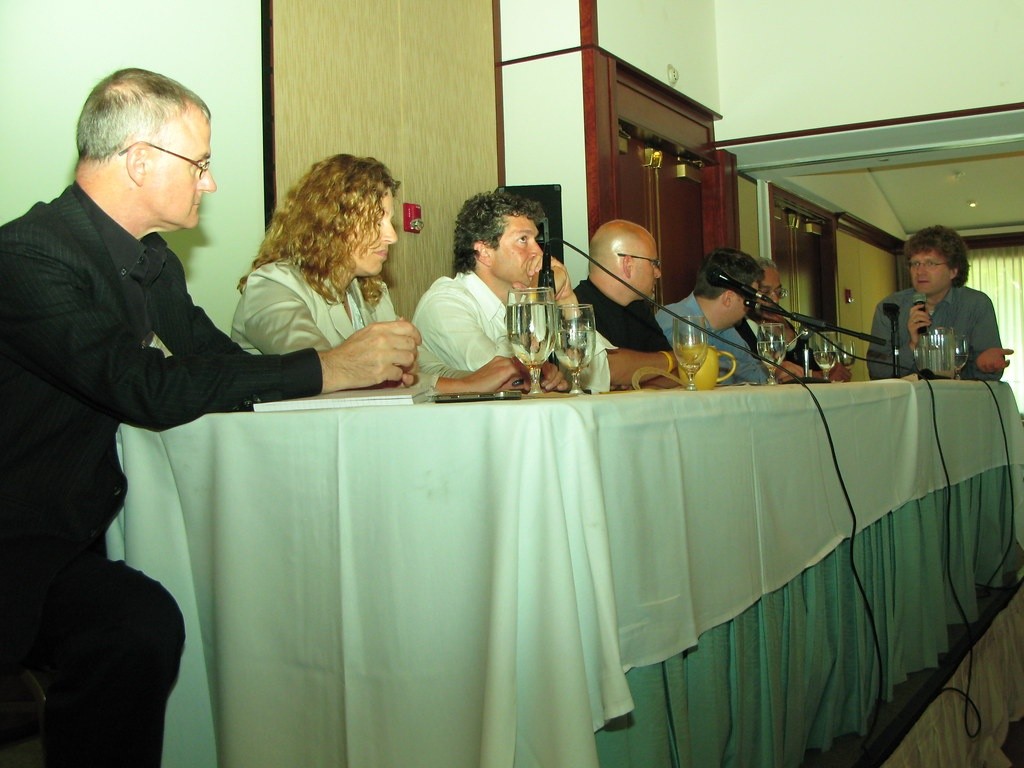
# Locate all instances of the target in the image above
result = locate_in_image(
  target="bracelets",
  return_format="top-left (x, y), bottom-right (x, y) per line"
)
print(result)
top-left (563, 312), bottom-right (585, 330)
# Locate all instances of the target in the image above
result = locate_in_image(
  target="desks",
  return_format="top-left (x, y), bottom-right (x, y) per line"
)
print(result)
top-left (105, 380), bottom-right (1024, 768)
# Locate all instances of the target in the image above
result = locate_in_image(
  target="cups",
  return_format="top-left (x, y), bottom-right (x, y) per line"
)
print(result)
top-left (913, 327), bottom-right (954, 379)
top-left (679, 345), bottom-right (735, 390)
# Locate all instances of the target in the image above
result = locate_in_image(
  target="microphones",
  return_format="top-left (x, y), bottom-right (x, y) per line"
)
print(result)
top-left (918, 368), bottom-right (952, 382)
top-left (912, 292), bottom-right (927, 334)
top-left (706, 266), bottom-right (770, 303)
top-left (537, 243), bottom-right (557, 305)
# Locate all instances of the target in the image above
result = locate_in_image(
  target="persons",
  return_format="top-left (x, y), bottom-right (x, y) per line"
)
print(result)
top-left (659, 351), bottom-right (674, 374)
top-left (866, 224), bottom-right (1014, 381)
top-left (655, 248), bottom-right (852, 389)
top-left (0, 66), bottom-right (423, 768)
top-left (572, 219), bottom-right (682, 390)
top-left (230, 154), bottom-right (568, 398)
top-left (410, 186), bottom-right (584, 403)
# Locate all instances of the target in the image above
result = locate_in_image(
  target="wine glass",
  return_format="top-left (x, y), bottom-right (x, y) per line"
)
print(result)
top-left (507, 287), bottom-right (558, 397)
top-left (757, 323), bottom-right (786, 386)
top-left (835, 341), bottom-right (856, 382)
top-left (673, 315), bottom-right (708, 391)
top-left (554, 304), bottom-right (596, 394)
top-left (954, 335), bottom-right (968, 380)
top-left (813, 332), bottom-right (839, 380)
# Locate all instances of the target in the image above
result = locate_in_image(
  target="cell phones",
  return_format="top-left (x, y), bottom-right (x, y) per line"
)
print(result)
top-left (435, 391), bottom-right (522, 403)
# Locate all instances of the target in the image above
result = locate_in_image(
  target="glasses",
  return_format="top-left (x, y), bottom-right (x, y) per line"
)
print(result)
top-left (756, 287), bottom-right (784, 298)
top-left (618, 253), bottom-right (663, 268)
top-left (118, 141), bottom-right (210, 180)
top-left (908, 258), bottom-right (946, 268)
